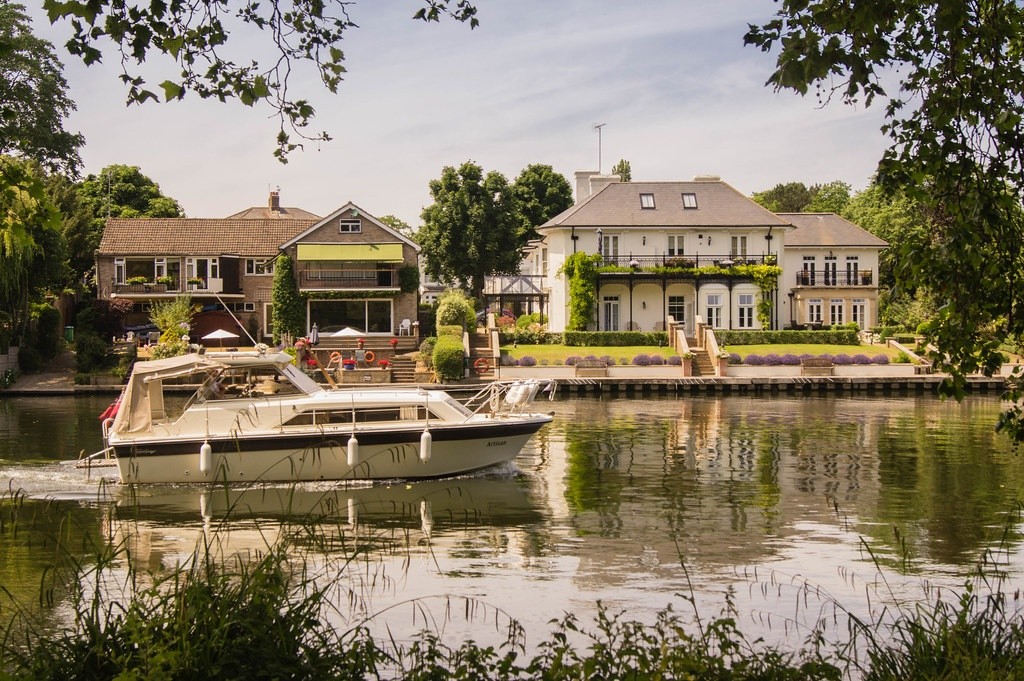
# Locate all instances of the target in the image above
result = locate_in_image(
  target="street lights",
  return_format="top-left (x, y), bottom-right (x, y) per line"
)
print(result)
top-left (594, 123), bottom-right (606, 174)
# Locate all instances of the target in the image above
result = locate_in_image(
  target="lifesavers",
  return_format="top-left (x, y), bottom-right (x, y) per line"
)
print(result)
top-left (474, 358), bottom-right (489, 373)
top-left (330, 351), bottom-right (341, 363)
top-left (364, 351), bottom-right (375, 363)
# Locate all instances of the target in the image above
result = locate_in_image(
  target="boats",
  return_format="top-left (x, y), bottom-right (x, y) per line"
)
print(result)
top-left (74, 292), bottom-right (558, 484)
top-left (106, 463), bottom-right (554, 529)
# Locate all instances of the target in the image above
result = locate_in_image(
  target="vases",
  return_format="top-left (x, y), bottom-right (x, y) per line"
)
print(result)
top-left (344, 364), bottom-right (354, 370)
top-left (393, 344), bottom-right (396, 350)
top-left (359, 343), bottom-right (364, 349)
top-left (382, 365), bottom-right (386, 369)
top-left (307, 366), bottom-right (316, 370)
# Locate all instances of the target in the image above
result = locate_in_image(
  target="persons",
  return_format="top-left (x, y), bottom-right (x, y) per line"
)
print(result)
top-left (203, 369), bottom-right (226, 399)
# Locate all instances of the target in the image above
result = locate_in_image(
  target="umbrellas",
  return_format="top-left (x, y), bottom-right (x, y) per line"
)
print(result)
top-left (201, 329), bottom-right (240, 352)
top-left (330, 327), bottom-right (366, 348)
top-left (310, 322), bottom-right (319, 355)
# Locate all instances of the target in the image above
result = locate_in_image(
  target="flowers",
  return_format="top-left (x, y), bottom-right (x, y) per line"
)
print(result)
top-left (390, 338), bottom-right (399, 344)
top-left (359, 338), bottom-right (366, 343)
top-left (378, 360), bottom-right (389, 365)
top-left (343, 358), bottom-right (358, 364)
top-left (307, 360), bottom-right (317, 365)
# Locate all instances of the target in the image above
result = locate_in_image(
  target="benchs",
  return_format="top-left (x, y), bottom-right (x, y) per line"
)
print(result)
top-left (575, 359), bottom-right (609, 377)
top-left (800, 358), bottom-right (835, 376)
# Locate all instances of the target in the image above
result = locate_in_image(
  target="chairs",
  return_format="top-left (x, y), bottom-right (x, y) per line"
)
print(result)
top-left (398, 319), bottom-right (411, 336)
top-left (148, 332), bottom-right (160, 347)
